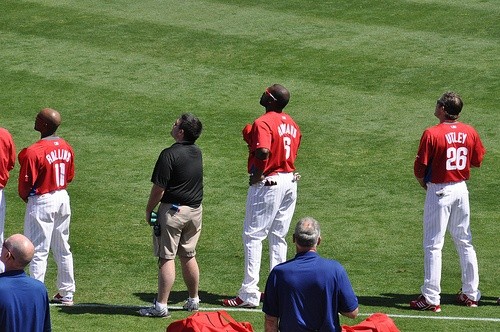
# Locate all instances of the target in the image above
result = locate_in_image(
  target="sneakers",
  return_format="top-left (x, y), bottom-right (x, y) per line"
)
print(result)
top-left (410, 293), bottom-right (442, 313)
top-left (136, 305), bottom-right (168, 317)
top-left (223, 296), bottom-right (257, 308)
top-left (454, 293), bottom-right (479, 308)
top-left (50, 293), bottom-right (74, 304)
top-left (182, 300), bottom-right (199, 312)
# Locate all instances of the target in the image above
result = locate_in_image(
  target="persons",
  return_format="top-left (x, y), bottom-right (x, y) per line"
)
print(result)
top-left (0, 234), bottom-right (51, 332)
top-left (216, 84), bottom-right (302, 310)
top-left (409, 89), bottom-right (486, 313)
top-left (18, 107), bottom-right (76, 307)
top-left (0, 126), bottom-right (16, 253)
top-left (138, 111), bottom-right (204, 319)
top-left (262, 216), bottom-right (359, 332)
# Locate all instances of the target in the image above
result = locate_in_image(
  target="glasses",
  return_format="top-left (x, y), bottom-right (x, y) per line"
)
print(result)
top-left (444, 111), bottom-right (460, 120)
top-left (150, 212), bottom-right (157, 226)
top-left (263, 87), bottom-right (278, 103)
top-left (2, 239), bottom-right (16, 260)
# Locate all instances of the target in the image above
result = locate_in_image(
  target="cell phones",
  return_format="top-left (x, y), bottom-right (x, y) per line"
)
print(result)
top-left (150, 210), bottom-right (157, 224)
